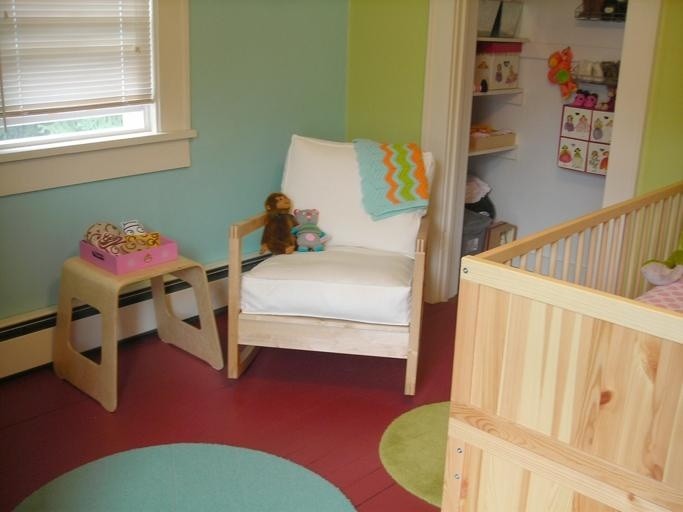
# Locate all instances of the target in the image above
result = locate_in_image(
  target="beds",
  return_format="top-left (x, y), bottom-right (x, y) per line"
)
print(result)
top-left (441, 181), bottom-right (683, 512)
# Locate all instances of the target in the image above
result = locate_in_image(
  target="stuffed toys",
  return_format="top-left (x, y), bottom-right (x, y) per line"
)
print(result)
top-left (640, 248), bottom-right (682, 286)
top-left (542, 46), bottom-right (577, 101)
top-left (258, 192), bottom-right (298, 256)
top-left (289, 207), bottom-right (332, 253)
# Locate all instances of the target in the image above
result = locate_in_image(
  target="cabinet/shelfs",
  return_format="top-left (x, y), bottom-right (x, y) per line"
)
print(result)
top-left (468, 37), bottom-right (527, 157)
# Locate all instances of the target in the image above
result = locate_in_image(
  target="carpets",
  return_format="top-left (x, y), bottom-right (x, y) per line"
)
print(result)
top-left (379, 401), bottom-right (451, 508)
top-left (12, 442), bottom-right (358, 512)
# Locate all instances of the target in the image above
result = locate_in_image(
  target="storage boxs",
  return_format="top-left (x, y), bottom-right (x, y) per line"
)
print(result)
top-left (473, 41), bottom-right (521, 90)
top-left (80, 236), bottom-right (177, 275)
top-left (461, 208), bottom-right (492, 256)
top-left (470, 124), bottom-right (515, 153)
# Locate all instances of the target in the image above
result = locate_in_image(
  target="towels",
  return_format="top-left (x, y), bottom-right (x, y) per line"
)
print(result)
top-left (351, 138), bottom-right (429, 222)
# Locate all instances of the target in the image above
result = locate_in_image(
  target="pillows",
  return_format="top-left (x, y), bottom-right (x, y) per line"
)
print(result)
top-left (280, 134), bottom-right (434, 259)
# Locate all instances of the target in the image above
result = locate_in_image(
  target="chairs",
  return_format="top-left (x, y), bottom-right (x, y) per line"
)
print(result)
top-left (226, 134), bottom-right (434, 397)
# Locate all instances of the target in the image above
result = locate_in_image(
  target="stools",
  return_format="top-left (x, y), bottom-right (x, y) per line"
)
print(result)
top-left (52, 255), bottom-right (224, 414)
